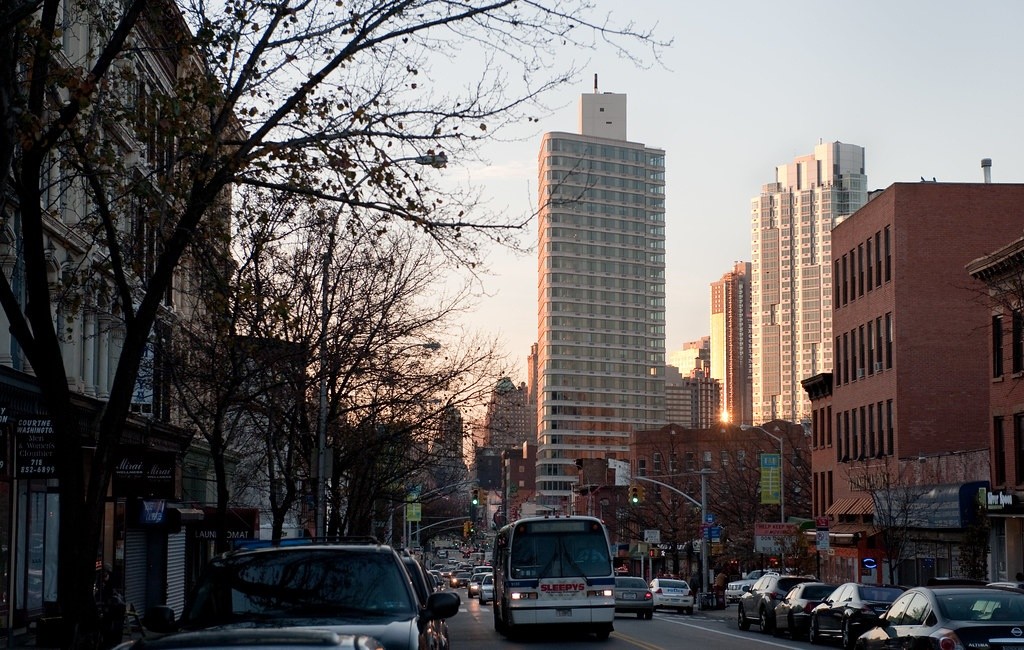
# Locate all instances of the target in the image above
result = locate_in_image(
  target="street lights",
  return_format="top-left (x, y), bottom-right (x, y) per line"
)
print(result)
top-left (570, 463), bottom-right (591, 516)
top-left (316, 154), bottom-right (448, 543)
top-left (740, 425), bottom-right (786, 575)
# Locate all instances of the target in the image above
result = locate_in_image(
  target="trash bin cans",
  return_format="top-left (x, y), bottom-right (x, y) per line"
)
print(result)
top-left (96, 603), bottom-right (126, 642)
top-left (697, 593), bottom-right (707, 610)
top-left (706, 592), bottom-right (716, 607)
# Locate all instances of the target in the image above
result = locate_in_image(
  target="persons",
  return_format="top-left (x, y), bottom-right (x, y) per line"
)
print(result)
top-left (656, 568), bottom-right (672, 578)
top-left (520, 540), bottom-right (548, 564)
top-left (713, 568), bottom-right (728, 609)
top-left (690, 573), bottom-right (699, 605)
top-left (1016, 572), bottom-right (1024, 582)
top-left (617, 563), bottom-right (629, 571)
top-left (74, 563), bottom-right (125, 650)
top-left (576, 539), bottom-right (606, 563)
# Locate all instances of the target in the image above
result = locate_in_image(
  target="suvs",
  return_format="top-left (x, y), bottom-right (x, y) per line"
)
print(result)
top-left (737, 572), bottom-right (820, 632)
top-left (130, 535), bottom-right (460, 649)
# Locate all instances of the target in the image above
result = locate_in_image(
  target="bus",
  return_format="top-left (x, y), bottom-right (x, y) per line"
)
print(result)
top-left (492, 514), bottom-right (616, 641)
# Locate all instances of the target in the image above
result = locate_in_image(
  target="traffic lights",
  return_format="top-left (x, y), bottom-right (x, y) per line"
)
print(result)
top-left (650, 548), bottom-right (658, 558)
top-left (628, 486), bottom-right (639, 503)
top-left (464, 489), bottom-right (489, 537)
top-left (639, 486), bottom-right (646, 502)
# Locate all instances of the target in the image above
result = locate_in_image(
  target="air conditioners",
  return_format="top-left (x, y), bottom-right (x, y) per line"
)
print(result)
top-left (874, 362), bottom-right (882, 373)
top-left (857, 369), bottom-right (865, 378)
top-left (132, 400), bottom-right (153, 416)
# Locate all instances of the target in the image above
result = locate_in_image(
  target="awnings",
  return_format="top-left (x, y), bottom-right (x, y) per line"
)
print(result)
top-left (824, 497), bottom-right (875, 514)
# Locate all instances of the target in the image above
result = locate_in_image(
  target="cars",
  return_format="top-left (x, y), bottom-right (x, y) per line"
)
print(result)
top-left (467, 573), bottom-right (487, 598)
top-left (771, 583), bottom-right (841, 640)
top-left (725, 570), bottom-right (770, 599)
top-left (437, 550), bottom-right (446, 559)
top-left (810, 582), bottom-right (1024, 650)
top-left (614, 576), bottom-right (654, 620)
top-left (423, 564), bottom-right (450, 593)
top-left (477, 574), bottom-right (495, 605)
top-left (649, 578), bottom-right (694, 615)
top-left (449, 558), bottom-right (493, 588)
